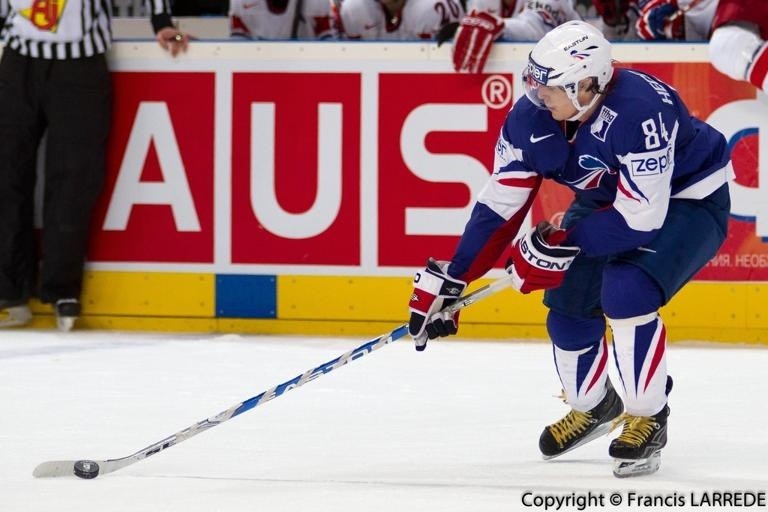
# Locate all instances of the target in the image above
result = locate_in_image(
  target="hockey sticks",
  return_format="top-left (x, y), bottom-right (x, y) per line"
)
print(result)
top-left (32, 275), bottom-right (513, 479)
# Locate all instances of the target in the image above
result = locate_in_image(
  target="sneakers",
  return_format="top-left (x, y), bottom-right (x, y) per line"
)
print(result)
top-left (51, 294), bottom-right (81, 314)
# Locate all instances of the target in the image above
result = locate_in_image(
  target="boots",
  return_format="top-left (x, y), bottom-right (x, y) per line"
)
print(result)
top-left (607, 376), bottom-right (674, 457)
top-left (539, 377), bottom-right (623, 455)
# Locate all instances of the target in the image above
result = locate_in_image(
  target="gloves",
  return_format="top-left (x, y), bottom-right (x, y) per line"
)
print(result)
top-left (504, 219), bottom-right (579, 295)
top-left (450, 10), bottom-right (503, 73)
top-left (407, 258), bottom-right (467, 351)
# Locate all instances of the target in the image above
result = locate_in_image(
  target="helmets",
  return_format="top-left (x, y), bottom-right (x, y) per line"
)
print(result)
top-left (526, 20), bottom-right (614, 94)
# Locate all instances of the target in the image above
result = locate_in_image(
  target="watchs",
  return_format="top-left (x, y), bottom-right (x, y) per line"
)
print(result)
top-left (169, 32), bottom-right (184, 43)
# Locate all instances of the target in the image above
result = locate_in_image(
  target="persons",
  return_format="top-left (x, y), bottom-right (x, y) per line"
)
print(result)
top-left (1, 1), bottom-right (193, 325)
top-left (403, 21), bottom-right (738, 461)
top-left (702, 0), bottom-right (767, 101)
top-left (225, 0), bottom-right (768, 43)
